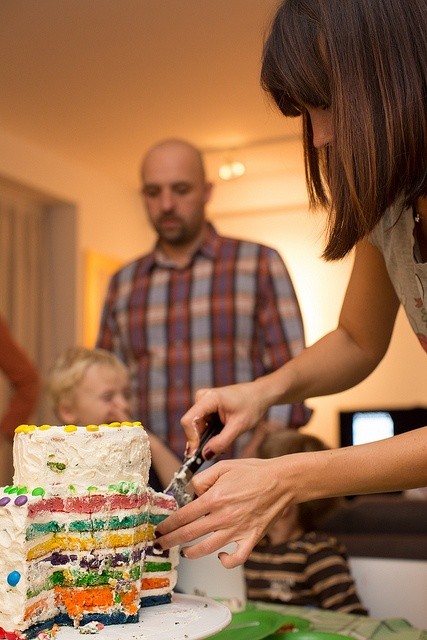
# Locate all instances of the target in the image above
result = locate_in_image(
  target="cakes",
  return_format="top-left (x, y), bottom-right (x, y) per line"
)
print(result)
top-left (0, 420), bottom-right (180, 636)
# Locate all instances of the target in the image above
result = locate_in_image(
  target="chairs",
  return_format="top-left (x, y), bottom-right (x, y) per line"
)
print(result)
top-left (345, 496), bottom-right (427, 560)
top-left (314, 498), bottom-right (349, 550)
top-left (347, 557), bottom-right (427, 630)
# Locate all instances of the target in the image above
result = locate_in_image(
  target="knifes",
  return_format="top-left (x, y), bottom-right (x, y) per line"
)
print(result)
top-left (161, 406), bottom-right (230, 531)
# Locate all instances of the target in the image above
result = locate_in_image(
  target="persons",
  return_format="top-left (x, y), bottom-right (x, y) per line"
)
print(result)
top-left (53, 345), bottom-right (132, 425)
top-left (243, 430), bottom-right (368, 616)
top-left (153, 0), bottom-right (426, 570)
top-left (96, 139), bottom-right (303, 493)
